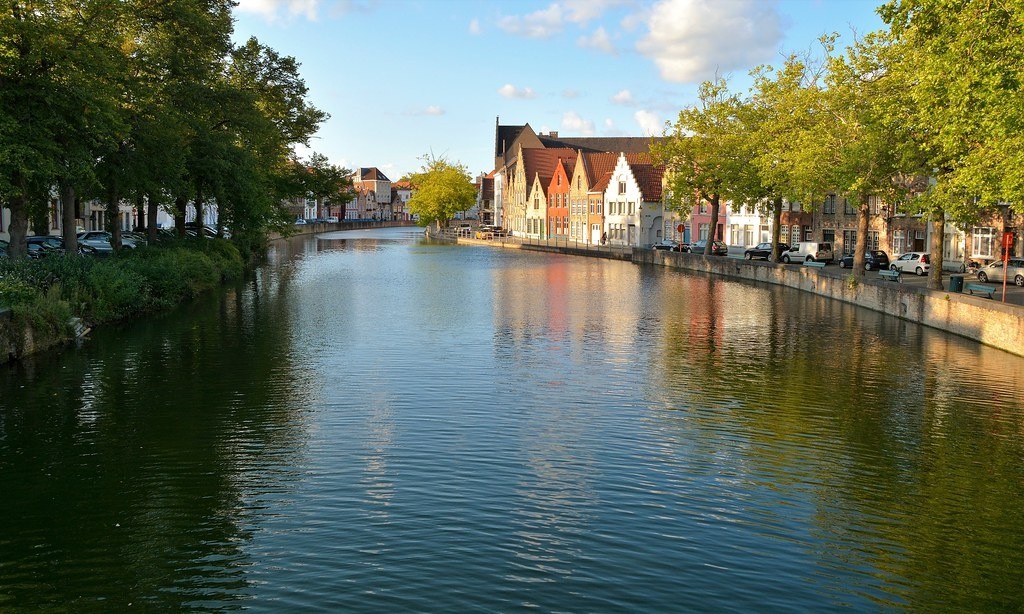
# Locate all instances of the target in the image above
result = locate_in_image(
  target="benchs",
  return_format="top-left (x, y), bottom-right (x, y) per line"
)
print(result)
top-left (878, 270), bottom-right (899, 281)
top-left (803, 261), bottom-right (825, 269)
top-left (965, 282), bottom-right (996, 299)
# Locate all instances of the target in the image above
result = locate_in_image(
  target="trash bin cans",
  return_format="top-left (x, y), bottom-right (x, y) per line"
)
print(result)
top-left (949, 275), bottom-right (963, 292)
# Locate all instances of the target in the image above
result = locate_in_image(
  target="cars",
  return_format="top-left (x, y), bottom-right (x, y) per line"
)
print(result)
top-left (0, 240), bottom-right (33, 264)
top-left (27, 221), bottom-right (232, 261)
top-left (975, 259), bottom-right (1024, 287)
top-left (295, 219), bottom-right (307, 225)
top-left (744, 242), bottom-right (790, 262)
top-left (453, 223), bottom-right (504, 239)
top-left (651, 240), bottom-right (680, 251)
top-left (837, 250), bottom-right (890, 271)
top-left (888, 252), bottom-right (931, 275)
top-left (685, 239), bottom-right (728, 255)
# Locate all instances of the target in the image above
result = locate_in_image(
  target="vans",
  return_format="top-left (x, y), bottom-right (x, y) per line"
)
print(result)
top-left (326, 216), bottom-right (339, 223)
top-left (780, 242), bottom-right (834, 264)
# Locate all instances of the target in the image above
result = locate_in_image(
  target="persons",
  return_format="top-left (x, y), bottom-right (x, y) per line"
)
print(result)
top-left (602, 232), bottom-right (608, 244)
